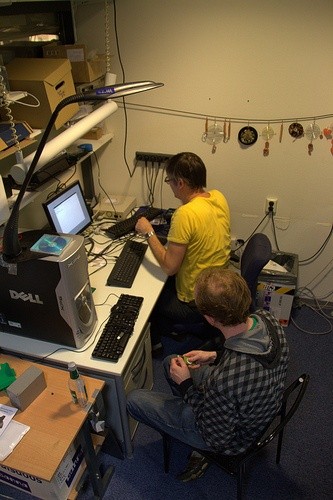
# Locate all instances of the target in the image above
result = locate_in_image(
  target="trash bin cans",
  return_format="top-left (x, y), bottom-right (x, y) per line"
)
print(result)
top-left (255, 251), bottom-right (299, 328)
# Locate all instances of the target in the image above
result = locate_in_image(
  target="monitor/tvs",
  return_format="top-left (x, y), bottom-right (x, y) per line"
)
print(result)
top-left (42, 180), bottom-right (93, 236)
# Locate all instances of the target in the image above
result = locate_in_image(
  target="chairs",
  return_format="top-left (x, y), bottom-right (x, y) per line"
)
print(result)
top-left (158, 373), bottom-right (309, 500)
top-left (170, 231), bottom-right (273, 359)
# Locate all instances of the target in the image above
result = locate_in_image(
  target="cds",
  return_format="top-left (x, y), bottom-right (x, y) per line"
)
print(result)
top-left (38, 235), bottom-right (67, 253)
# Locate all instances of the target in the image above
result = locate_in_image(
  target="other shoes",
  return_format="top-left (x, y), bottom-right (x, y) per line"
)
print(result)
top-left (167, 327), bottom-right (180, 337)
top-left (151, 341), bottom-right (161, 350)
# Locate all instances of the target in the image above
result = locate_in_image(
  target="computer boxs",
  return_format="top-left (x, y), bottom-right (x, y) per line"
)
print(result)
top-left (0, 230), bottom-right (99, 349)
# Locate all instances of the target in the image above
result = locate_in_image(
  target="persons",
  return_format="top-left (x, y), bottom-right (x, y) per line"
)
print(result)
top-left (135, 153), bottom-right (232, 351)
top-left (126, 267), bottom-right (289, 483)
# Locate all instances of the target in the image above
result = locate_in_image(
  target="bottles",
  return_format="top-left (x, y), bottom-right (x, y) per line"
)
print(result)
top-left (68, 361), bottom-right (88, 406)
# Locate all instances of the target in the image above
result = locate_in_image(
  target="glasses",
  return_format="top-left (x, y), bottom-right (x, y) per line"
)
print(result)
top-left (165, 176), bottom-right (173, 185)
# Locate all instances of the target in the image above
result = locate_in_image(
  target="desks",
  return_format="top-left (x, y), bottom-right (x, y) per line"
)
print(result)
top-left (0, 209), bottom-right (169, 459)
top-left (0, 353), bottom-right (116, 500)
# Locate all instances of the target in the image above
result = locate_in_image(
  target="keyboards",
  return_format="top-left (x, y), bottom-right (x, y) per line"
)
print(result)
top-left (107, 239), bottom-right (148, 288)
top-left (105, 206), bottom-right (163, 240)
top-left (92, 294), bottom-right (143, 362)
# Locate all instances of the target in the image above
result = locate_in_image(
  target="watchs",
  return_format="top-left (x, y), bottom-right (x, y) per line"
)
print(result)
top-left (145, 232), bottom-right (154, 239)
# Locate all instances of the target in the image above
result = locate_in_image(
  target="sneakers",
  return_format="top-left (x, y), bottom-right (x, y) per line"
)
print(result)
top-left (178, 453), bottom-right (210, 484)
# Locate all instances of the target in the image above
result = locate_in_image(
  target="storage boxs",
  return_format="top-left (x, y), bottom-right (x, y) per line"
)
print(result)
top-left (0, 41), bottom-right (116, 152)
top-left (0, 436), bottom-right (85, 500)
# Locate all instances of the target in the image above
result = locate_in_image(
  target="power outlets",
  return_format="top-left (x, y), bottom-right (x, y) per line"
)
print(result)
top-left (265, 199), bottom-right (277, 213)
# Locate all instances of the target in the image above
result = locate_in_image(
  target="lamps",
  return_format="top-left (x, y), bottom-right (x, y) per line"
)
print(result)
top-left (2, 80), bottom-right (164, 265)
